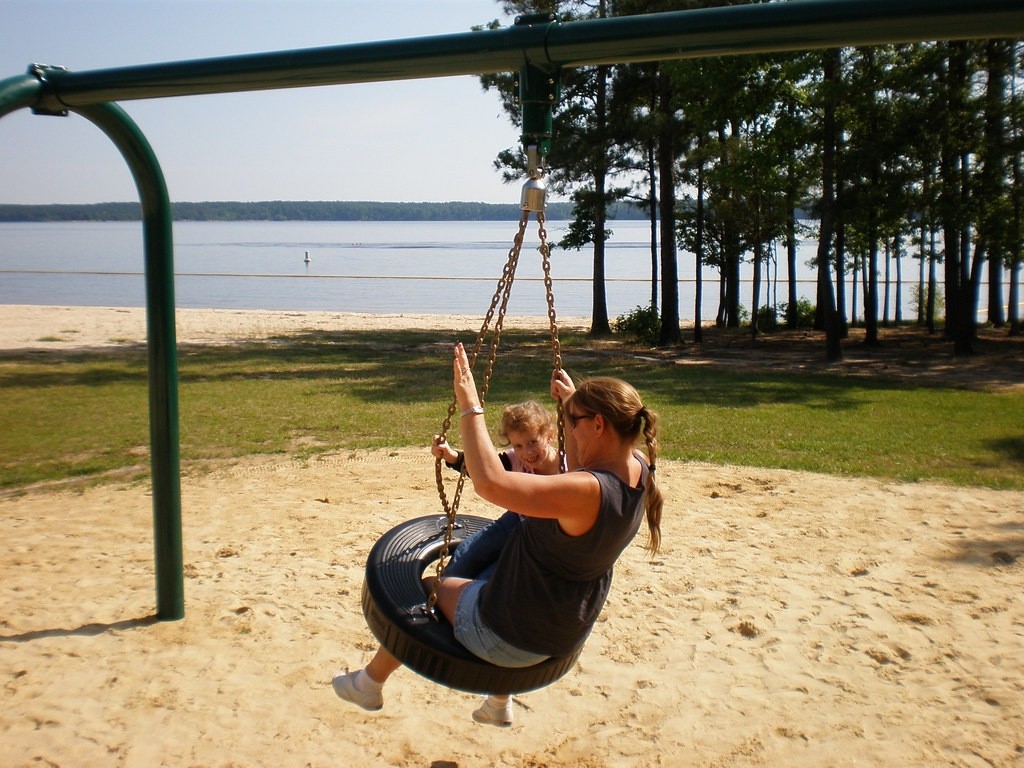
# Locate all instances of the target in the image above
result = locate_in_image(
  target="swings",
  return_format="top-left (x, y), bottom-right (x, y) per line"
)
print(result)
top-left (359, 143), bottom-right (586, 701)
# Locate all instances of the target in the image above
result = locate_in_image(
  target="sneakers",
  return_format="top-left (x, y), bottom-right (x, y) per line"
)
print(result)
top-left (472, 699), bottom-right (512, 727)
top-left (332, 670), bottom-right (383, 711)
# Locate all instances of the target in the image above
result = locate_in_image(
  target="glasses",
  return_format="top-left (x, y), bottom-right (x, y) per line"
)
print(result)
top-left (568, 414), bottom-right (608, 429)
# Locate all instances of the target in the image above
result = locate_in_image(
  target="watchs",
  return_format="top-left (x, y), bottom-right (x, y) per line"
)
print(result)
top-left (460, 405), bottom-right (484, 418)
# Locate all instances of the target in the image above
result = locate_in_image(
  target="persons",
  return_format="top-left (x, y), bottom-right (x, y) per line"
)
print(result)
top-left (432, 400), bottom-right (568, 581)
top-left (332, 339), bottom-right (663, 728)
